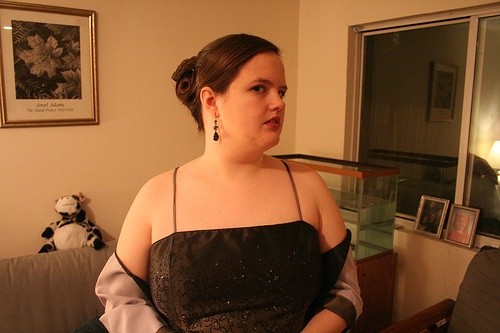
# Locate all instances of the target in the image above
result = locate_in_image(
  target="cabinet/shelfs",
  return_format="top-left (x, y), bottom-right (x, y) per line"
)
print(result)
top-left (350, 239), bottom-right (398, 333)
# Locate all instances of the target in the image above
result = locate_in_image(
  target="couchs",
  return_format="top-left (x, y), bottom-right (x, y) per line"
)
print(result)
top-left (0, 240), bottom-right (119, 333)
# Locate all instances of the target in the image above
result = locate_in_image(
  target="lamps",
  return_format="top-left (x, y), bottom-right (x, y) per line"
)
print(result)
top-left (487, 141), bottom-right (500, 176)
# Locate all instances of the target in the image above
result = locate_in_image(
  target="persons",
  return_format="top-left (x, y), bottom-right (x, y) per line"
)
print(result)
top-left (446, 212), bottom-right (470, 244)
top-left (95, 32), bottom-right (364, 333)
top-left (418, 201), bottom-right (439, 233)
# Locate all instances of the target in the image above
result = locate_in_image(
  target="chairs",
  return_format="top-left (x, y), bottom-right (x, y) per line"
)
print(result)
top-left (377, 245), bottom-right (500, 333)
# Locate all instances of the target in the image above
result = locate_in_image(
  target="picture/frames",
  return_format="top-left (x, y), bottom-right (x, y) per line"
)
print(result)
top-left (0, 0), bottom-right (100, 128)
top-left (443, 204), bottom-right (481, 248)
top-left (413, 195), bottom-right (451, 239)
top-left (426, 60), bottom-right (459, 123)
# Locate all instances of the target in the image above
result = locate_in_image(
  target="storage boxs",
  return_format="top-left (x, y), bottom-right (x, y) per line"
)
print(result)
top-left (271, 154), bottom-right (400, 263)
top-left (367, 150), bottom-right (463, 217)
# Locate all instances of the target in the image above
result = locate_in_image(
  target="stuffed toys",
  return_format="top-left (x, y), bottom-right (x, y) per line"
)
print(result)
top-left (38, 192), bottom-right (105, 253)
